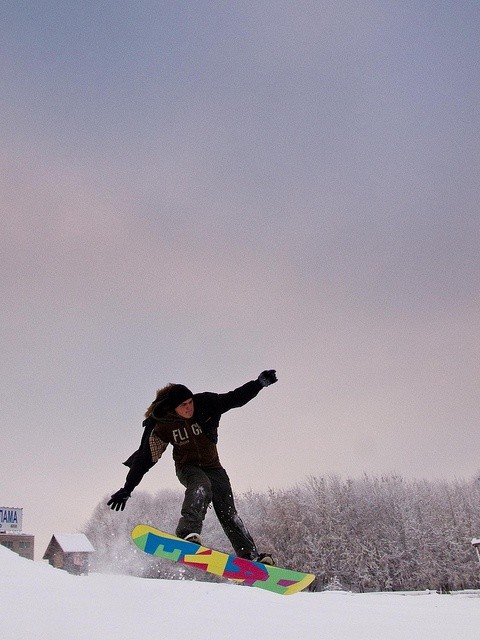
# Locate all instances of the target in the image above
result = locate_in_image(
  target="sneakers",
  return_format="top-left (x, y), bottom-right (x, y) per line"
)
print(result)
top-left (252, 553), bottom-right (274, 566)
top-left (184, 533), bottom-right (203, 545)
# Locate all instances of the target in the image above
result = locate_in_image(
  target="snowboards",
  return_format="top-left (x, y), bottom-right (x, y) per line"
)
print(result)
top-left (131, 524), bottom-right (315, 595)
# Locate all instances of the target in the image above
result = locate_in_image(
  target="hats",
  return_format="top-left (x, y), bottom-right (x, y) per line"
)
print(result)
top-left (167, 384), bottom-right (194, 410)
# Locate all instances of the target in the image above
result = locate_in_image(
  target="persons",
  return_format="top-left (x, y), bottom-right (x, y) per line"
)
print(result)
top-left (107, 368), bottom-right (279, 566)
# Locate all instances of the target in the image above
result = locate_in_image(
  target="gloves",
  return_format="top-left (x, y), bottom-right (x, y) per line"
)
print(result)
top-left (106, 488), bottom-right (131, 511)
top-left (258, 369), bottom-right (278, 387)
